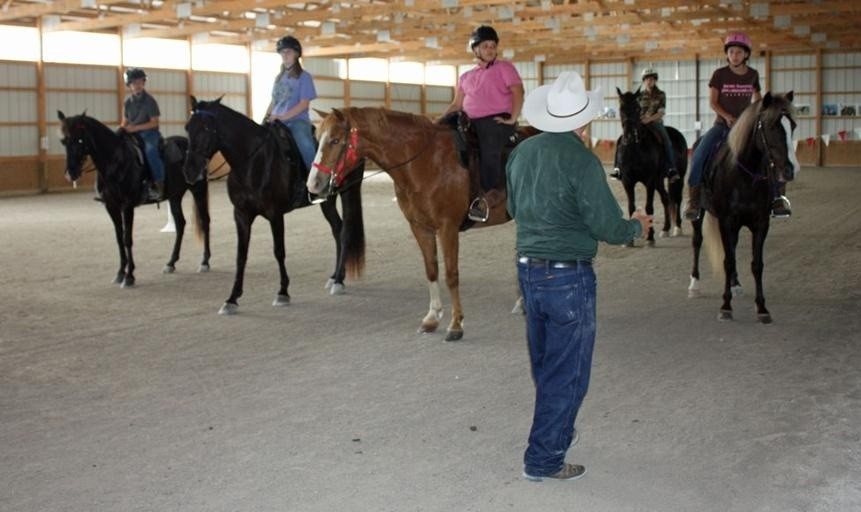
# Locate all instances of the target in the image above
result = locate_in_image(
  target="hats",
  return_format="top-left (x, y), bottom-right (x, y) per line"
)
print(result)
top-left (523, 72), bottom-right (605, 133)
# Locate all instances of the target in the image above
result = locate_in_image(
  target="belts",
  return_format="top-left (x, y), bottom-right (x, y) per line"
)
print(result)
top-left (517, 254), bottom-right (594, 269)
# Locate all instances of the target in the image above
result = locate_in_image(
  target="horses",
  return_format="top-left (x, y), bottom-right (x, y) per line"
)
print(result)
top-left (613, 86), bottom-right (690, 248)
top-left (181, 91), bottom-right (368, 317)
top-left (56, 107), bottom-right (211, 291)
top-left (304, 104), bottom-right (544, 345)
top-left (686, 89), bottom-right (797, 326)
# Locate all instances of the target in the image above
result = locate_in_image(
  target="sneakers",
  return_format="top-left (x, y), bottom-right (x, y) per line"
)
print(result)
top-left (669, 169), bottom-right (681, 184)
top-left (610, 168), bottom-right (621, 176)
top-left (469, 189), bottom-right (506, 217)
top-left (149, 181), bottom-right (164, 200)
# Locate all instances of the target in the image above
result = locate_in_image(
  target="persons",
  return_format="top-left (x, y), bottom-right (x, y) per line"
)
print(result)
top-left (261, 36), bottom-right (317, 173)
top-left (95, 67), bottom-right (166, 202)
top-left (682, 32), bottom-right (792, 220)
top-left (608, 66), bottom-right (680, 184)
top-left (505, 70), bottom-right (658, 483)
top-left (433, 24), bottom-right (524, 213)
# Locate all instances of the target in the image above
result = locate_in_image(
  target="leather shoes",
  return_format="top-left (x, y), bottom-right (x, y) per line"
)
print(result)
top-left (570, 430), bottom-right (579, 446)
top-left (523, 463), bottom-right (585, 482)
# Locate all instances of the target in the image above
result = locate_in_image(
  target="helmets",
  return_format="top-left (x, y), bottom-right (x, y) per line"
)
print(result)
top-left (724, 33), bottom-right (752, 55)
top-left (469, 26), bottom-right (498, 47)
top-left (123, 67), bottom-right (145, 86)
top-left (276, 36), bottom-right (301, 58)
top-left (641, 68), bottom-right (658, 81)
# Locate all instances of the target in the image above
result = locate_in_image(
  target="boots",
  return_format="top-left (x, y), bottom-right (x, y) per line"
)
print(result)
top-left (686, 186), bottom-right (702, 219)
top-left (774, 183), bottom-right (791, 214)
